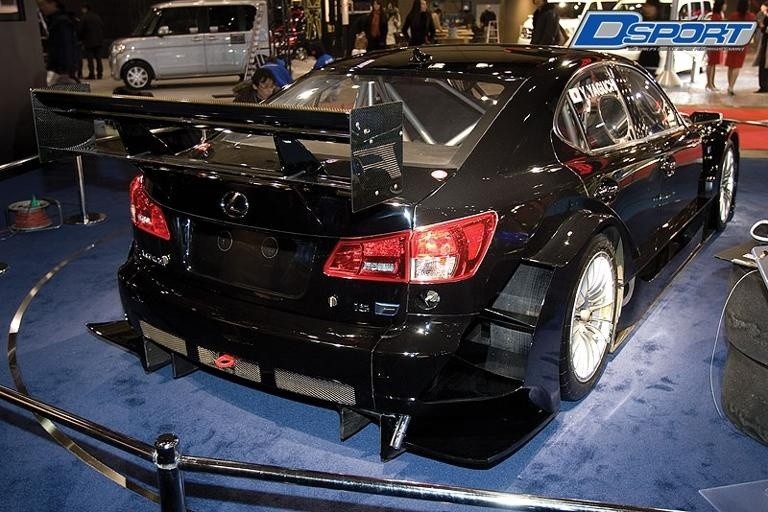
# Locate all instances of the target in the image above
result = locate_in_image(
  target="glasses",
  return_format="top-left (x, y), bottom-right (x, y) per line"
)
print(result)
top-left (259, 83), bottom-right (276, 90)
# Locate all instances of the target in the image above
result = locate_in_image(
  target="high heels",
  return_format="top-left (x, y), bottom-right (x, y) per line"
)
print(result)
top-left (728, 89), bottom-right (736, 96)
top-left (706, 84), bottom-right (719, 91)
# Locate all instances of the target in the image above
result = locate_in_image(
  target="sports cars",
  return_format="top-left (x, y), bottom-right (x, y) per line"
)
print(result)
top-left (29, 44), bottom-right (739, 470)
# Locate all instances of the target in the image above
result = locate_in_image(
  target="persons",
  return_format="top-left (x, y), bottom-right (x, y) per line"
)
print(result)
top-left (231, 5), bottom-right (335, 103)
top-left (344, 0), bottom-right (497, 58)
top-left (638, 0), bottom-right (768, 97)
top-left (36, 0), bottom-right (108, 80)
top-left (530, 0), bottom-right (561, 45)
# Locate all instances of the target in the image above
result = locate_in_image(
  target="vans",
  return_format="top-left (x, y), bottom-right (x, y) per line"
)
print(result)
top-left (518, 0), bottom-right (715, 78)
top-left (109, 0), bottom-right (270, 90)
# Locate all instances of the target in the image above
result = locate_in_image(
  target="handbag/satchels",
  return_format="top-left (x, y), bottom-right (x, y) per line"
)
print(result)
top-left (424, 32), bottom-right (434, 43)
top-left (393, 26), bottom-right (411, 44)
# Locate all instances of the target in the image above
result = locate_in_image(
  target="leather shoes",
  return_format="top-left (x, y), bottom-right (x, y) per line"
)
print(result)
top-left (755, 88), bottom-right (768, 93)
top-left (77, 73), bottom-right (102, 79)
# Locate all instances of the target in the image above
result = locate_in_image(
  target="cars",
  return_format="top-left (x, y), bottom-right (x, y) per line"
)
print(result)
top-left (273, 21), bottom-right (316, 61)
